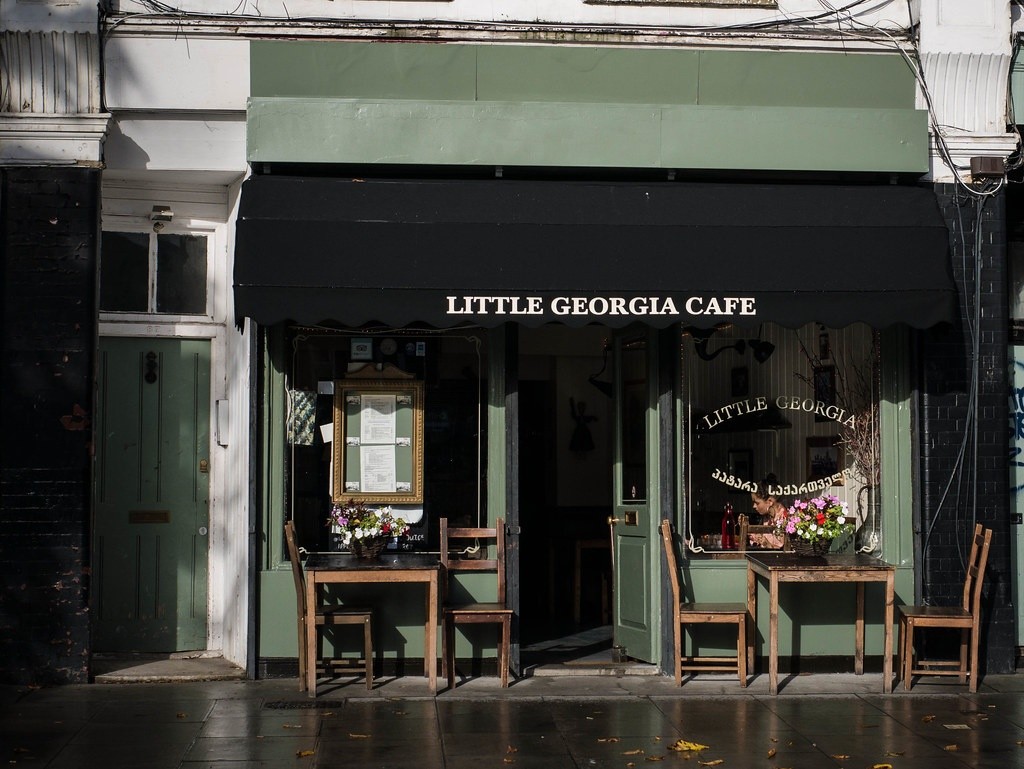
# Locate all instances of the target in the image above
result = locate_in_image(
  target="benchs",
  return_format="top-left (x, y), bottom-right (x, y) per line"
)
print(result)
top-left (685, 510), bottom-right (763, 544)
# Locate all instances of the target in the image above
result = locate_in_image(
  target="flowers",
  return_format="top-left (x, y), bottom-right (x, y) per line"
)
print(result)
top-left (793, 329), bottom-right (881, 483)
top-left (772, 495), bottom-right (855, 542)
top-left (326, 498), bottom-right (410, 544)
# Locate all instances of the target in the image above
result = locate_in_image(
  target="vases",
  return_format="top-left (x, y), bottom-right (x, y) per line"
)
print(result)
top-left (855, 484), bottom-right (881, 559)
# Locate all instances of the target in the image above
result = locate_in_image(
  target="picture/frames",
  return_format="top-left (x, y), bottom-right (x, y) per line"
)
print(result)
top-left (332, 380), bottom-right (423, 505)
top-left (814, 365), bottom-right (838, 421)
top-left (727, 450), bottom-right (752, 492)
top-left (806, 437), bottom-right (844, 487)
top-left (730, 368), bottom-right (749, 395)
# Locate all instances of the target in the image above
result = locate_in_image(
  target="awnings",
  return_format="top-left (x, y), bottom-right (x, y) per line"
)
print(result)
top-left (233, 176), bottom-right (958, 329)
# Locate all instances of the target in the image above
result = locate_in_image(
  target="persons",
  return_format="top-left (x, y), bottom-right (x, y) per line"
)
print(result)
top-left (738, 472), bottom-right (789, 549)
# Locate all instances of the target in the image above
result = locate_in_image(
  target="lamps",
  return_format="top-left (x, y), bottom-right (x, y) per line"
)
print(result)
top-left (749, 323), bottom-right (774, 362)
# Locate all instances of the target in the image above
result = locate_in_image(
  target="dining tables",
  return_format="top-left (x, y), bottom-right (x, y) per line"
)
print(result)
top-left (744, 554), bottom-right (895, 694)
top-left (548, 532), bottom-right (611, 624)
top-left (305, 554), bottom-right (438, 698)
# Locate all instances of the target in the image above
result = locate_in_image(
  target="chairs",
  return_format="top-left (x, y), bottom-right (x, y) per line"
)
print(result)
top-left (662, 519), bottom-right (747, 688)
top-left (594, 569), bottom-right (610, 625)
top-left (285, 520), bottom-right (373, 693)
top-left (898, 523), bottom-right (992, 693)
top-left (440, 518), bottom-right (513, 688)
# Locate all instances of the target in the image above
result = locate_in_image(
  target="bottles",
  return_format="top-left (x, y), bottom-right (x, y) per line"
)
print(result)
top-left (720, 501), bottom-right (736, 551)
